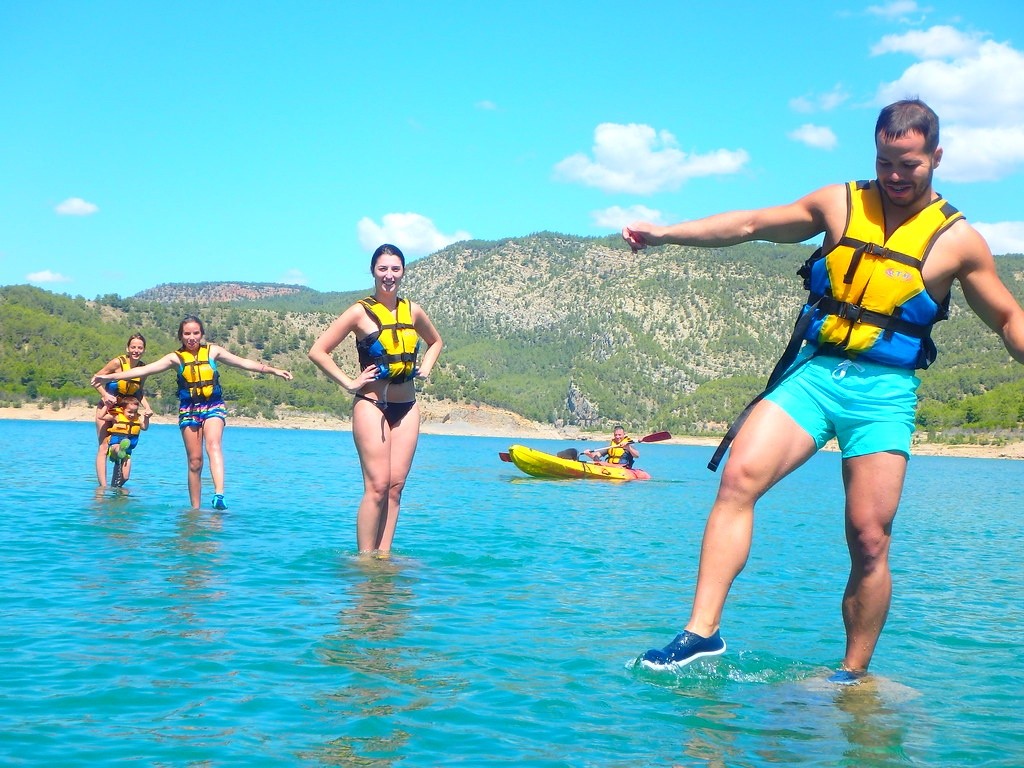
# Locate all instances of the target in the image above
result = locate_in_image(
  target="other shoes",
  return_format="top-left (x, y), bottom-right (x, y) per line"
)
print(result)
top-left (126, 449), bottom-right (131, 458)
top-left (360, 554), bottom-right (397, 574)
top-left (827, 669), bottom-right (862, 684)
top-left (643, 628), bottom-right (726, 670)
top-left (118, 439), bottom-right (130, 458)
top-left (212, 493), bottom-right (227, 510)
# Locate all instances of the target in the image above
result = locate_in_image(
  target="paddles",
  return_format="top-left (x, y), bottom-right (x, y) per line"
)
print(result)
top-left (499, 451), bottom-right (602, 464)
top-left (581, 431), bottom-right (672, 455)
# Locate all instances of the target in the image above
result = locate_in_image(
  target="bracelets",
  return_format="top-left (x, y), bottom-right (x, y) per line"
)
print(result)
top-left (262, 365), bottom-right (265, 373)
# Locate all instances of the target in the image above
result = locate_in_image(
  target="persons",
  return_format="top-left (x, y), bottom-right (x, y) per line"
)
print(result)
top-left (91, 332), bottom-right (154, 487)
top-left (307, 244), bottom-right (443, 560)
top-left (91, 316), bottom-right (293, 511)
top-left (621, 99), bottom-right (1024, 685)
top-left (584, 426), bottom-right (640, 469)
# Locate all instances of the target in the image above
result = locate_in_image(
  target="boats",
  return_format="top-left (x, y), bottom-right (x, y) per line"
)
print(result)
top-left (509, 444), bottom-right (652, 482)
